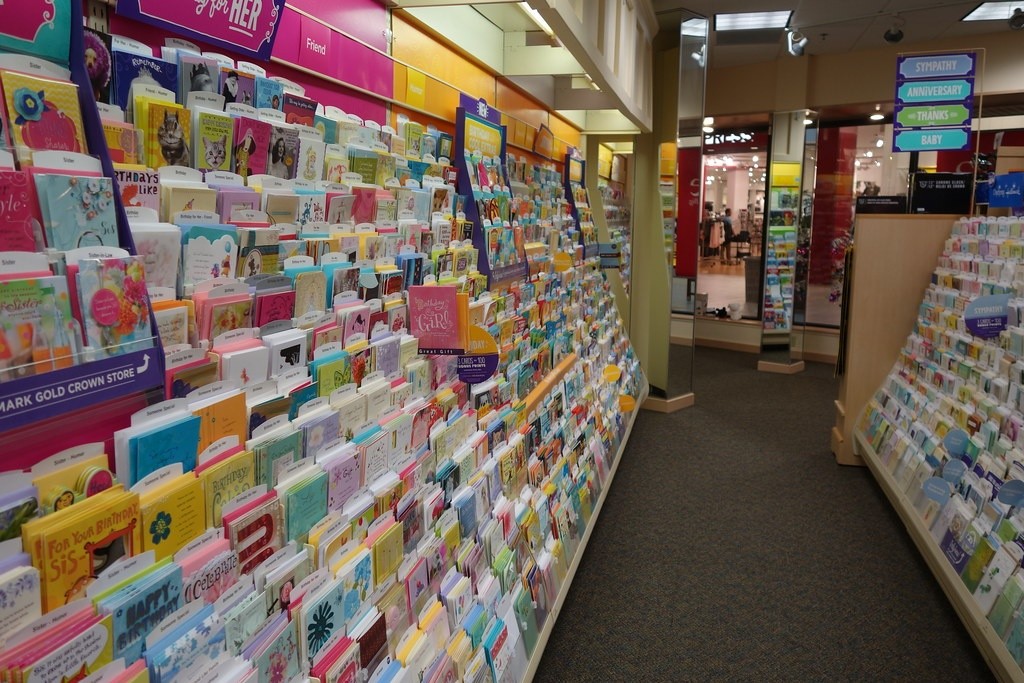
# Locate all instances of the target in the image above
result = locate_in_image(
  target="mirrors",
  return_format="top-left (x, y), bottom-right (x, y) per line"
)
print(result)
top-left (631, 9), bottom-right (709, 399)
top-left (760, 109), bottom-right (823, 365)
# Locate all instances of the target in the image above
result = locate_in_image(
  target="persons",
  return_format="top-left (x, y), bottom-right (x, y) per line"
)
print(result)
top-left (720, 208), bottom-right (733, 265)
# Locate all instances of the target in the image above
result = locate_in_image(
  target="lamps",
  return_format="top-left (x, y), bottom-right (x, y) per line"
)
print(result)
top-left (883, 13), bottom-right (906, 44)
top-left (1009, 7), bottom-right (1024, 32)
top-left (691, 43), bottom-right (706, 68)
top-left (786, 28), bottom-right (808, 58)
